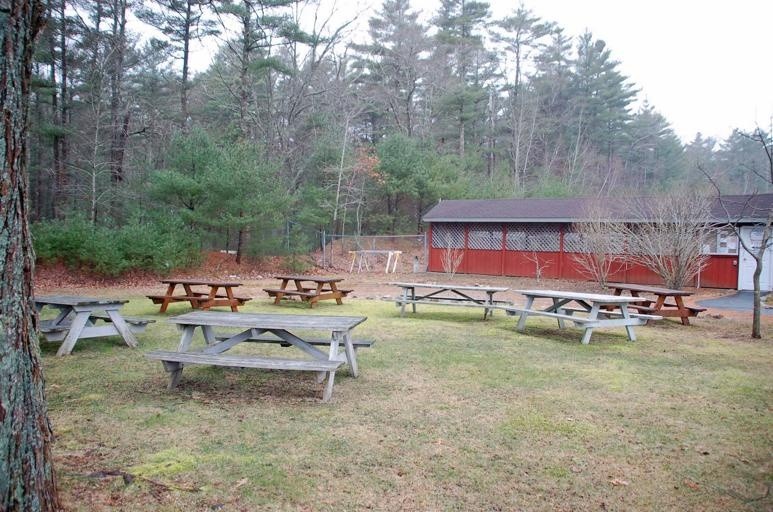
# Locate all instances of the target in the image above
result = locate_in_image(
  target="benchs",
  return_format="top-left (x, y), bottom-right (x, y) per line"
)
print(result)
top-left (261, 287), bottom-right (354, 297)
top-left (603, 298), bottom-right (708, 313)
top-left (144, 291), bottom-right (253, 303)
top-left (142, 333), bottom-right (376, 373)
top-left (38, 315), bottom-right (156, 334)
top-left (504, 307), bottom-right (664, 325)
top-left (396, 293), bottom-right (514, 312)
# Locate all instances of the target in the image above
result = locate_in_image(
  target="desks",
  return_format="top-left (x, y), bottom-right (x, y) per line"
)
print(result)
top-left (158, 279), bottom-right (243, 313)
top-left (166, 308), bottom-right (367, 402)
top-left (34, 295), bottom-right (140, 358)
top-left (273, 276), bottom-right (344, 307)
top-left (512, 289), bottom-right (646, 345)
top-left (393, 281), bottom-right (509, 320)
top-left (603, 281), bottom-right (695, 327)
